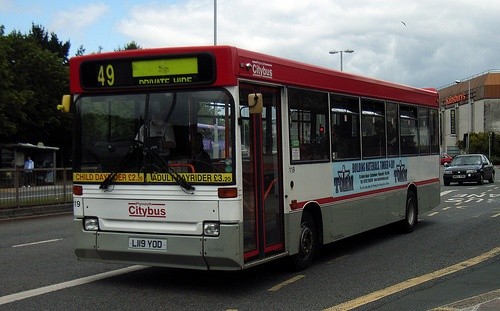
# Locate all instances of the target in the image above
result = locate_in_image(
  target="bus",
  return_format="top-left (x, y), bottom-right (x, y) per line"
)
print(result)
top-left (56, 45), bottom-right (441, 271)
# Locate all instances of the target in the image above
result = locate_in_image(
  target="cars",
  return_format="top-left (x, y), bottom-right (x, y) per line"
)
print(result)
top-left (440, 152), bottom-right (452, 167)
top-left (443, 154), bottom-right (496, 186)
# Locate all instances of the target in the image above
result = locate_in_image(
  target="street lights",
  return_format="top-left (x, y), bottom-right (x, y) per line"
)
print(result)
top-left (488, 130), bottom-right (494, 161)
top-left (329, 49), bottom-right (355, 71)
top-left (454, 80), bottom-right (471, 154)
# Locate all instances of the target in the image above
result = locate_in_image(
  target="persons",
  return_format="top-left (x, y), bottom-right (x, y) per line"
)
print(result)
top-left (21, 156), bottom-right (34, 188)
top-left (189, 132), bottom-right (211, 171)
top-left (135, 101), bottom-right (174, 143)
top-left (332, 119), bottom-right (399, 158)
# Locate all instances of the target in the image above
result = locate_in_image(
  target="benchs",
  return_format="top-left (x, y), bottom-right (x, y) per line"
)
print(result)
top-left (402, 135), bottom-right (418, 153)
